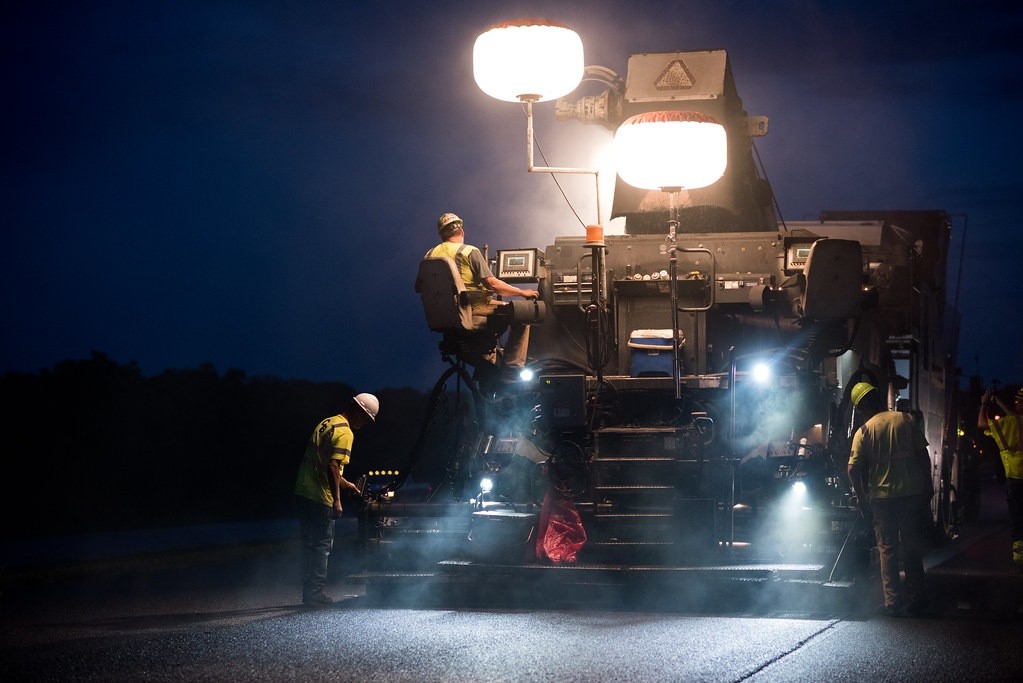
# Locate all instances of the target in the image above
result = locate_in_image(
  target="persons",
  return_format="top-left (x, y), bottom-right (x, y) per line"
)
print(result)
top-left (977, 384), bottom-right (1023, 561)
top-left (293, 393), bottom-right (379, 608)
top-left (847, 382), bottom-right (935, 618)
top-left (415, 211), bottom-right (540, 385)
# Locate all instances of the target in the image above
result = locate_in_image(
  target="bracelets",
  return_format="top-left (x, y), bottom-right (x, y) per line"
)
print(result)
top-left (982, 402), bottom-right (988, 405)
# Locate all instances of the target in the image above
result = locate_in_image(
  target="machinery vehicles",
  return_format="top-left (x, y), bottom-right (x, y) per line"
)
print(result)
top-left (353, 15), bottom-right (981, 606)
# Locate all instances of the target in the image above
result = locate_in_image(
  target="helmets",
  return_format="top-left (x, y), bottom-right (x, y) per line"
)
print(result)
top-left (850, 381), bottom-right (876, 407)
top-left (353, 392), bottom-right (379, 423)
top-left (438, 212), bottom-right (463, 233)
top-left (1014, 391), bottom-right (1023, 399)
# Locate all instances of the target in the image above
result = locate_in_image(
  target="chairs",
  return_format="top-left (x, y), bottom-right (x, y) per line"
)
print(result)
top-left (769, 238), bottom-right (863, 349)
top-left (417, 257), bottom-right (498, 357)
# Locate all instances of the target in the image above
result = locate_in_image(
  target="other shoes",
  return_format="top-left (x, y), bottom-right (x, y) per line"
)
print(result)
top-left (501, 357), bottom-right (533, 379)
top-left (886, 595), bottom-right (904, 616)
top-left (1012, 540), bottom-right (1023, 560)
top-left (299, 598), bottom-right (337, 613)
